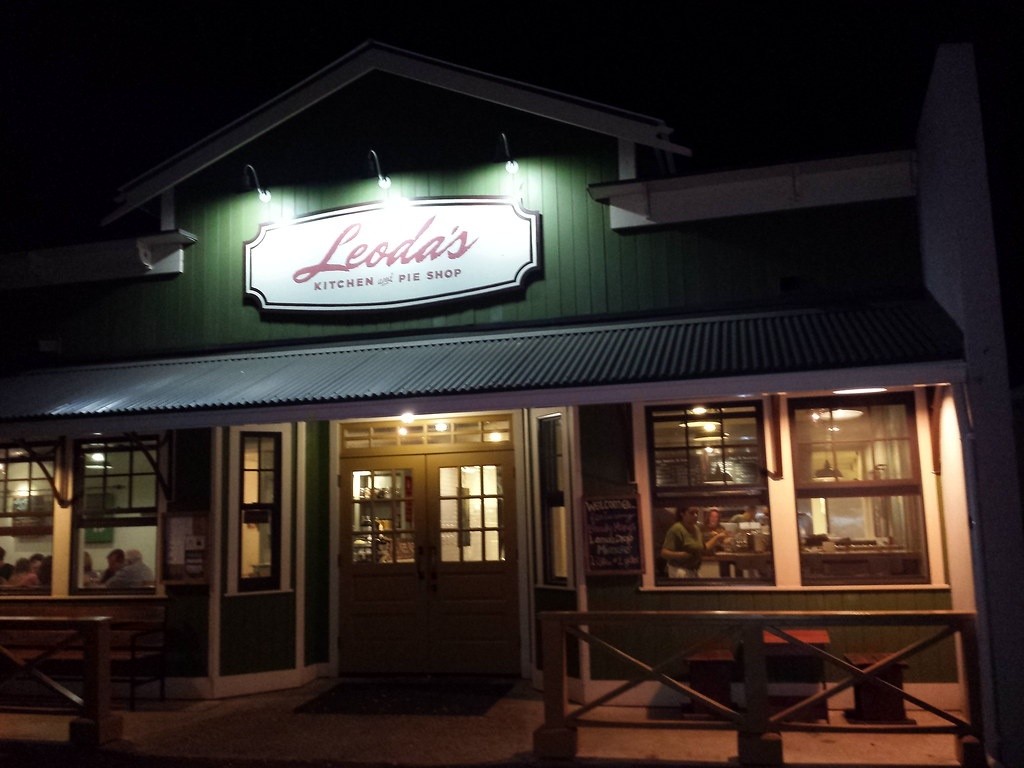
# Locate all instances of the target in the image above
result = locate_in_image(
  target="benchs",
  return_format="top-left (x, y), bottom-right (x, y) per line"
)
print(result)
top-left (0, 595), bottom-right (171, 713)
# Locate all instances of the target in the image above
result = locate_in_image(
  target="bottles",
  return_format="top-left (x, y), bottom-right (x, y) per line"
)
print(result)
top-left (731, 531), bottom-right (771, 553)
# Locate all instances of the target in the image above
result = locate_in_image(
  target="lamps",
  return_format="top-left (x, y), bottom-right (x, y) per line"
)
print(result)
top-left (244, 164), bottom-right (272, 202)
top-left (813, 419), bottom-right (837, 480)
top-left (367, 148), bottom-right (392, 189)
top-left (495, 131), bottom-right (519, 173)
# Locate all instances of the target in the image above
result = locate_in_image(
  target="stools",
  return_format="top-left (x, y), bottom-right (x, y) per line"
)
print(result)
top-left (682, 649), bottom-right (744, 720)
top-left (846, 652), bottom-right (909, 721)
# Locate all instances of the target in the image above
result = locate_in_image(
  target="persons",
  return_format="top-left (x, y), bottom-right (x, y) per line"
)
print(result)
top-left (0, 544), bottom-right (151, 594)
top-left (661, 505), bottom-right (767, 579)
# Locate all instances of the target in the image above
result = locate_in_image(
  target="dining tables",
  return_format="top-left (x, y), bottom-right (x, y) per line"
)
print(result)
top-left (714, 536), bottom-right (919, 581)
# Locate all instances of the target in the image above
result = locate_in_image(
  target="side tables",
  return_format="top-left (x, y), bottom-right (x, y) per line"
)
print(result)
top-left (762, 627), bottom-right (831, 722)
